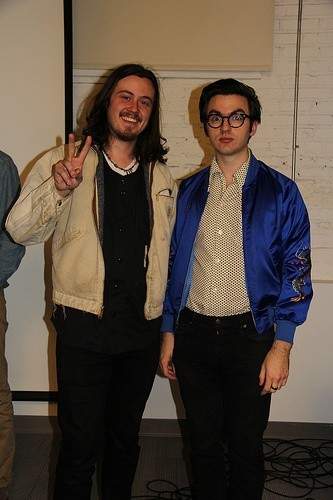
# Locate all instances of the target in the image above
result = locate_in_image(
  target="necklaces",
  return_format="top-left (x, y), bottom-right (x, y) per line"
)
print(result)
top-left (103, 147), bottom-right (139, 175)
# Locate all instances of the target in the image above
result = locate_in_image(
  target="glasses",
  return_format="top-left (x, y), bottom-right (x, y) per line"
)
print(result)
top-left (206, 112), bottom-right (250, 128)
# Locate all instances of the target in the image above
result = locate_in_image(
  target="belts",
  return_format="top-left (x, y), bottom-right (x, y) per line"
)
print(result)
top-left (180, 307), bottom-right (255, 336)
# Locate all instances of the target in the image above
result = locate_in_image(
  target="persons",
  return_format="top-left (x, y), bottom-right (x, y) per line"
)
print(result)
top-left (5, 60), bottom-right (179, 500)
top-left (1, 151), bottom-right (27, 500)
top-left (154, 78), bottom-right (315, 500)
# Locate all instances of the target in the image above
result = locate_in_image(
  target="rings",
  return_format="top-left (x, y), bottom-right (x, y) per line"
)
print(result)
top-left (271, 385), bottom-right (278, 391)
